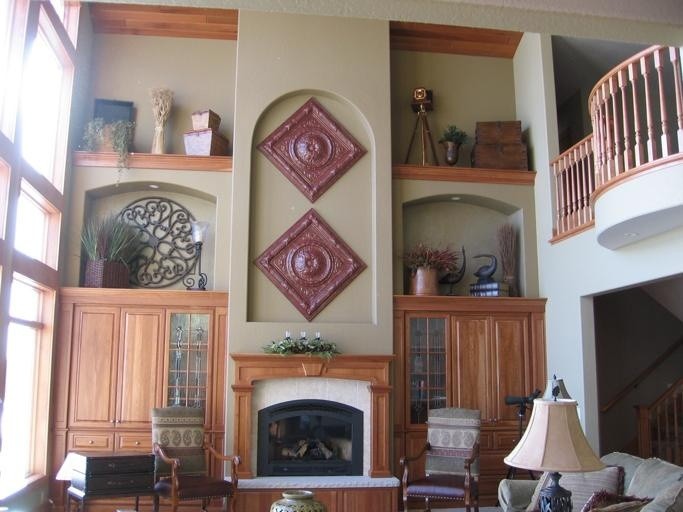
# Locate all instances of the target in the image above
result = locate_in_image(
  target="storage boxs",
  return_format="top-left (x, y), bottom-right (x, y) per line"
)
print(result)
top-left (471, 141), bottom-right (530, 170)
top-left (188, 107), bottom-right (222, 130)
top-left (183, 130), bottom-right (228, 159)
top-left (472, 118), bottom-right (522, 143)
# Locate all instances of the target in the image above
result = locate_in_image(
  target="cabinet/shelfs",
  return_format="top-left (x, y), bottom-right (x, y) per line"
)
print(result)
top-left (167, 291), bottom-right (223, 474)
top-left (55, 286), bottom-right (166, 498)
top-left (391, 295), bottom-right (452, 497)
top-left (64, 450), bottom-right (161, 512)
top-left (453, 294), bottom-right (545, 475)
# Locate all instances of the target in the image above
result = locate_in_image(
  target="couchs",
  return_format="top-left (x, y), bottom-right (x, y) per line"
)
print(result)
top-left (495, 448), bottom-right (682, 512)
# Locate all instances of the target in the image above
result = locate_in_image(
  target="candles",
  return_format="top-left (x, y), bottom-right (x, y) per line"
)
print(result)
top-left (187, 218), bottom-right (209, 243)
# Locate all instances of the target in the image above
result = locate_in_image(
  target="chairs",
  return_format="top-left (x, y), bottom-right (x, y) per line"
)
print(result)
top-left (147, 405), bottom-right (243, 512)
top-left (400, 406), bottom-right (483, 512)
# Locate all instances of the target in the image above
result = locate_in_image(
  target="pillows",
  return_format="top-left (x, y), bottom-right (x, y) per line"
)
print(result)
top-left (557, 464), bottom-right (621, 512)
top-left (580, 488), bottom-right (652, 512)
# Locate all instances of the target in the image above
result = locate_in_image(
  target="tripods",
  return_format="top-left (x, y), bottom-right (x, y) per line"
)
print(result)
top-left (496, 418), bottom-right (534, 506)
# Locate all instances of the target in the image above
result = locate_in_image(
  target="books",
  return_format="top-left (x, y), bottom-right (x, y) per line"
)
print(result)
top-left (468, 282), bottom-right (510, 297)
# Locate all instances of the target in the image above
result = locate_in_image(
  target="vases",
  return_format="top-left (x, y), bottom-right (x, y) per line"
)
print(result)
top-left (500, 273), bottom-right (520, 297)
top-left (268, 490), bottom-right (326, 512)
top-left (410, 265), bottom-right (444, 295)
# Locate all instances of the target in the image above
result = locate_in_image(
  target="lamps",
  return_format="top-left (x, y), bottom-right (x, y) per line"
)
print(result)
top-left (540, 374), bottom-right (572, 400)
top-left (503, 396), bottom-right (607, 512)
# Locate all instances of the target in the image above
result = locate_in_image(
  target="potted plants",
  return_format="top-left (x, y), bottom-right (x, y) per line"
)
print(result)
top-left (435, 121), bottom-right (468, 168)
top-left (81, 118), bottom-right (144, 189)
top-left (152, 83), bottom-right (175, 155)
top-left (79, 210), bottom-right (145, 290)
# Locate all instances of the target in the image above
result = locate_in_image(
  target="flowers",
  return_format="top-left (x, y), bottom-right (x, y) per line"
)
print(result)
top-left (403, 242), bottom-right (460, 271)
